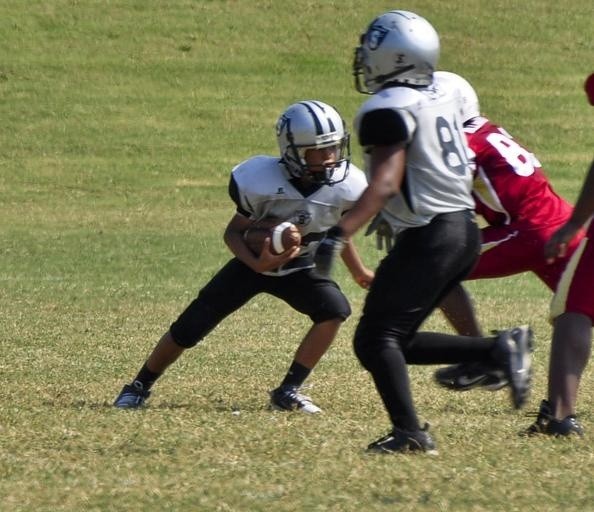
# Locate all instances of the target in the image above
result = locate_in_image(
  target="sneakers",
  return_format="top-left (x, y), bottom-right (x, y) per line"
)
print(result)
top-left (267, 386), bottom-right (323, 416)
top-left (113, 383), bottom-right (151, 411)
top-left (490, 324), bottom-right (533, 410)
top-left (518, 398), bottom-right (585, 440)
top-left (367, 422), bottom-right (440, 457)
top-left (434, 360), bottom-right (509, 391)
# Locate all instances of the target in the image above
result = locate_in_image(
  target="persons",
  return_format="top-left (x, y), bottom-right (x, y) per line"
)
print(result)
top-left (518, 73), bottom-right (594, 437)
top-left (433, 115), bottom-right (586, 390)
top-left (309, 10), bottom-right (534, 454)
top-left (112, 100), bottom-right (375, 417)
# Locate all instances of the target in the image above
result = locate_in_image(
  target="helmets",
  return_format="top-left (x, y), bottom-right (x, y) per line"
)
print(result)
top-left (431, 71), bottom-right (480, 124)
top-left (352, 10), bottom-right (440, 96)
top-left (275, 99), bottom-right (352, 186)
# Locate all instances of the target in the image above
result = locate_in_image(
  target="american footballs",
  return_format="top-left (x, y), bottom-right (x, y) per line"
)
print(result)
top-left (241, 219), bottom-right (302, 255)
